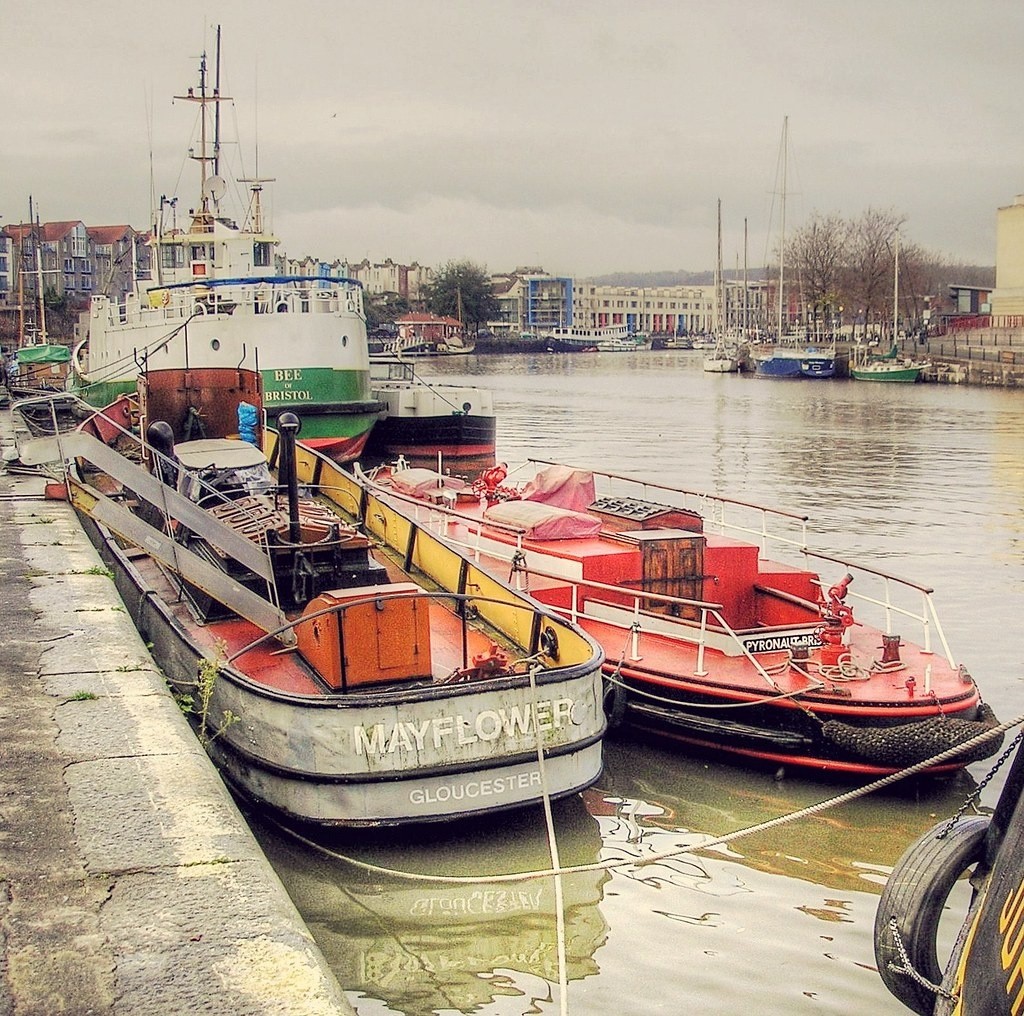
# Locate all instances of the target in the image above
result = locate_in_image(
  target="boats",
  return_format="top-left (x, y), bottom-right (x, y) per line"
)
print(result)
top-left (70, 369), bottom-right (605, 828)
top-left (69, 22), bottom-right (388, 436)
top-left (371, 356), bottom-right (495, 456)
top-left (664, 338), bottom-right (689, 350)
top-left (351, 451), bottom-right (1005, 772)
top-left (596, 340), bottom-right (638, 351)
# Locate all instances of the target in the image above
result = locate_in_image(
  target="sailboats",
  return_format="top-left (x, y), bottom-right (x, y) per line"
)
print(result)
top-left (4, 195), bottom-right (74, 400)
top-left (436, 287), bottom-right (476, 354)
top-left (701, 113), bottom-right (933, 382)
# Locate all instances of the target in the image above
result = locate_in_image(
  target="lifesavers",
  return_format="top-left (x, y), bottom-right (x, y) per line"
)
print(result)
top-left (871, 813), bottom-right (994, 1016)
